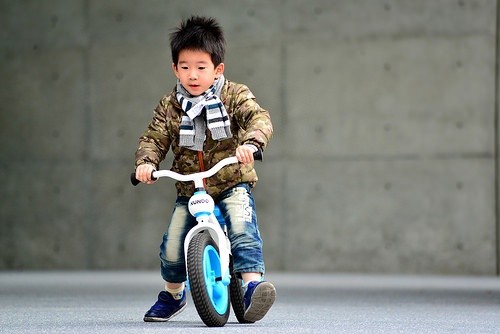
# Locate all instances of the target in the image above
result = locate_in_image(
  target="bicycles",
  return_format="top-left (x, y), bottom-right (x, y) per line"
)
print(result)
top-left (130, 148), bottom-right (255, 328)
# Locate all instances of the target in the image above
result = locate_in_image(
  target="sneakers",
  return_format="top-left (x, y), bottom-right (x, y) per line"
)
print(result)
top-left (242, 281), bottom-right (277, 323)
top-left (143, 287), bottom-right (188, 322)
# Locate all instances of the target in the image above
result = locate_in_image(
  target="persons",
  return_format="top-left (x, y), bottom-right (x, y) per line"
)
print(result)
top-left (135, 15), bottom-right (277, 322)
